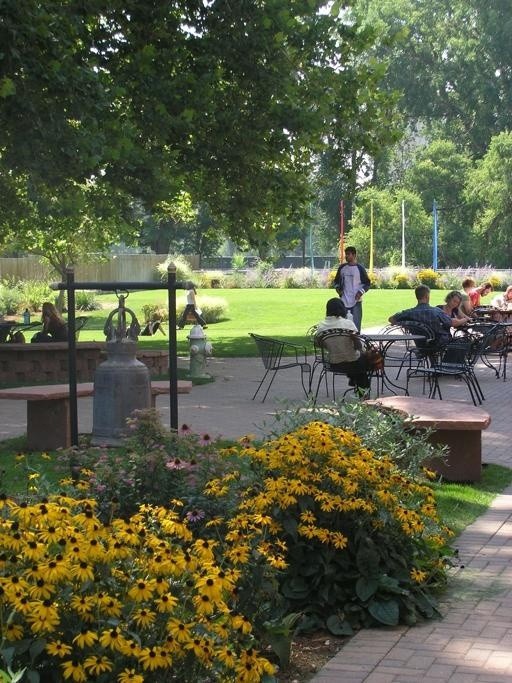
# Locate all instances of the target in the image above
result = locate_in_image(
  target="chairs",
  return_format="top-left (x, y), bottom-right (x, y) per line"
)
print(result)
top-left (248, 305), bottom-right (511, 410)
top-left (5, 316), bottom-right (87, 344)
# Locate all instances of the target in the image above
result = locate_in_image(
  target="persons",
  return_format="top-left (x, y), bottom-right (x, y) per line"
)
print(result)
top-left (388, 279), bottom-right (512, 376)
top-left (331, 247), bottom-right (370, 335)
top-left (32, 302), bottom-right (69, 341)
top-left (315, 298), bottom-right (371, 400)
top-left (176, 280), bottom-right (208, 329)
top-left (141, 314), bottom-right (166, 336)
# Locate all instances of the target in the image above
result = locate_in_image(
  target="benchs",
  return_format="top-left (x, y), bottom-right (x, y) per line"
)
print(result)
top-left (1, 380), bottom-right (193, 448)
top-left (362, 394), bottom-right (489, 482)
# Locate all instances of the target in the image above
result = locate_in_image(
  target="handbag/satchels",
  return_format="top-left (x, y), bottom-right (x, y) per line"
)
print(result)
top-left (361, 349), bottom-right (383, 370)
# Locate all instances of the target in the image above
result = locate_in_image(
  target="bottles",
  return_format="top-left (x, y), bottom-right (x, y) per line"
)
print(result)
top-left (24, 308), bottom-right (30, 325)
top-left (346, 310), bottom-right (353, 322)
